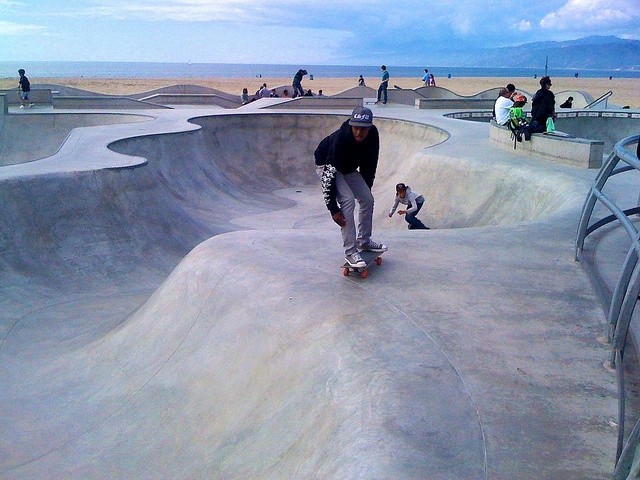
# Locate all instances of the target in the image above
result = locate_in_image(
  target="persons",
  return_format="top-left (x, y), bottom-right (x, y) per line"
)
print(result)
top-left (18, 69), bottom-right (35, 109)
top-left (531, 76), bottom-right (556, 129)
top-left (270, 89), bottom-right (279, 97)
top-left (389, 183), bottom-right (428, 229)
top-left (281, 90), bottom-right (290, 98)
top-left (305, 90), bottom-right (313, 96)
top-left (493, 84), bottom-right (517, 117)
top-left (429, 74), bottom-right (435, 86)
top-left (494, 87), bottom-right (528, 126)
top-left (259, 83), bottom-right (271, 97)
top-left (292, 69), bottom-right (308, 98)
top-left (314, 108), bottom-right (387, 267)
top-left (359, 75), bottom-right (364, 86)
top-left (252, 86), bottom-right (263, 101)
top-left (560, 97), bottom-right (573, 109)
top-left (375, 65), bottom-right (389, 104)
top-left (422, 69), bottom-right (430, 86)
top-left (317, 90), bottom-right (324, 96)
top-left (241, 88), bottom-right (248, 104)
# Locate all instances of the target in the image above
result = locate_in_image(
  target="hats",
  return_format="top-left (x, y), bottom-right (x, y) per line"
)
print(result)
top-left (396, 183), bottom-right (405, 191)
top-left (513, 94), bottom-right (528, 103)
top-left (349, 107), bottom-right (373, 126)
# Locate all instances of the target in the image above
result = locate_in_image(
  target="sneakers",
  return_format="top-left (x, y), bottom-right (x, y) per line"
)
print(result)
top-left (358, 239), bottom-right (388, 252)
top-left (345, 250), bottom-right (367, 268)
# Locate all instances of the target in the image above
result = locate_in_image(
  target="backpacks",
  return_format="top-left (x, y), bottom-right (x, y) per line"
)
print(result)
top-left (516, 120), bottom-right (545, 142)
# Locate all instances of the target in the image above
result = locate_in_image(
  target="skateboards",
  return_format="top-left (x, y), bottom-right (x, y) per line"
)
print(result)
top-left (340, 250), bottom-right (384, 278)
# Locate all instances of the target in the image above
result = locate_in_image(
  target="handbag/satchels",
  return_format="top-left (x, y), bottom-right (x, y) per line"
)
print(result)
top-left (546, 118), bottom-right (555, 132)
